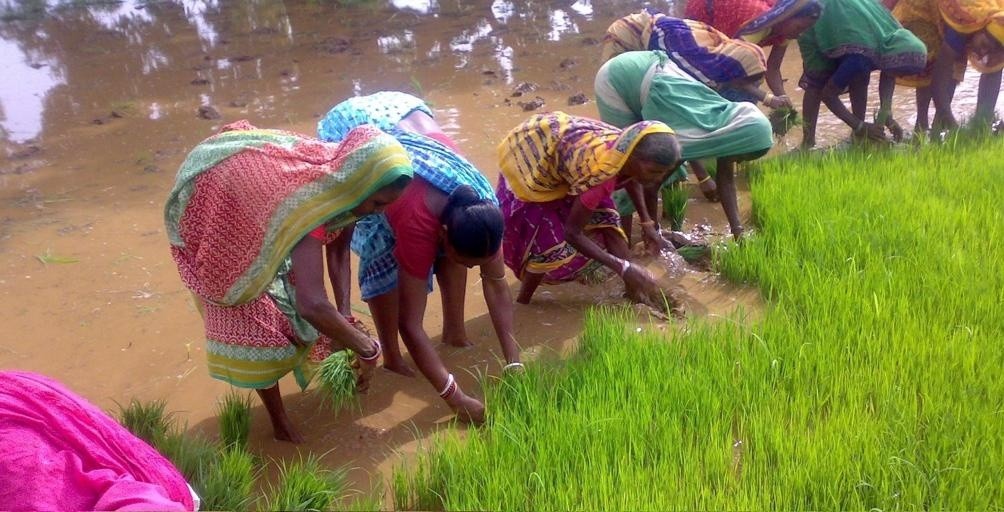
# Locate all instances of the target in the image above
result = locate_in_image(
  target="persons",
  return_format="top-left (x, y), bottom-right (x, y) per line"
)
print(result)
top-left (1, 367), bottom-right (205, 512)
top-left (593, 48), bottom-right (776, 249)
top-left (494, 108), bottom-right (685, 319)
top-left (600, 7), bottom-right (771, 203)
top-left (682, 0), bottom-right (824, 135)
top-left (881, 0), bottom-right (1003, 145)
top-left (313, 89), bottom-right (526, 432)
top-left (159, 117), bottom-right (416, 447)
top-left (792, 0), bottom-right (929, 151)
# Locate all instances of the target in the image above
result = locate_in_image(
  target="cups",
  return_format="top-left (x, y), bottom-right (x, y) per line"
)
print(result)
top-left (357, 339), bottom-right (382, 378)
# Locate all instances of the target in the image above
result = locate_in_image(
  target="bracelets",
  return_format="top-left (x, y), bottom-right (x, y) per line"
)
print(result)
top-left (778, 94), bottom-right (790, 99)
top-left (654, 223), bottom-right (662, 235)
top-left (438, 372), bottom-right (459, 403)
top-left (852, 119), bottom-right (864, 137)
top-left (699, 175), bottom-right (711, 185)
top-left (357, 338), bottom-right (385, 364)
top-left (619, 259), bottom-right (632, 279)
top-left (762, 91), bottom-right (774, 107)
top-left (500, 361), bottom-right (526, 373)
top-left (729, 224), bottom-right (746, 234)
top-left (637, 219), bottom-right (656, 227)
